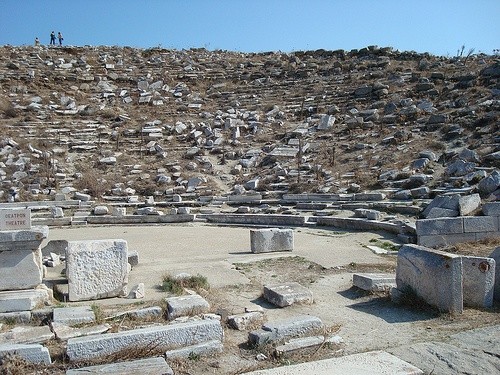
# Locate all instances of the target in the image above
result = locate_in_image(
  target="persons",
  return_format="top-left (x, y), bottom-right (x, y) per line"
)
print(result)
top-left (59, 31), bottom-right (64, 45)
top-left (50, 30), bottom-right (57, 45)
top-left (36, 36), bottom-right (41, 46)
